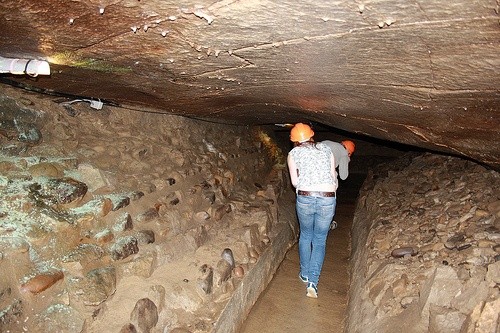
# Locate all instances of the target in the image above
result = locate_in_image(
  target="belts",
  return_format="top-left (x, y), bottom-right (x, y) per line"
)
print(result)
top-left (297, 190), bottom-right (336, 198)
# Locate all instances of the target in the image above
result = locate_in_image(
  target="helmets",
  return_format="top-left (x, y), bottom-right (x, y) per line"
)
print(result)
top-left (290, 122), bottom-right (314, 143)
top-left (342, 141), bottom-right (355, 154)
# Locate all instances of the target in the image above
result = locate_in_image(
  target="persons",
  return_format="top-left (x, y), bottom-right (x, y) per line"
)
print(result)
top-left (286, 123), bottom-right (337, 299)
top-left (319, 140), bottom-right (356, 231)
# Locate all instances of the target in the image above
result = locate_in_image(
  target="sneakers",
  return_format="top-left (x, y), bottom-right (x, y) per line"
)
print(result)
top-left (329, 221), bottom-right (337, 230)
top-left (306, 282), bottom-right (318, 299)
top-left (299, 272), bottom-right (309, 282)
